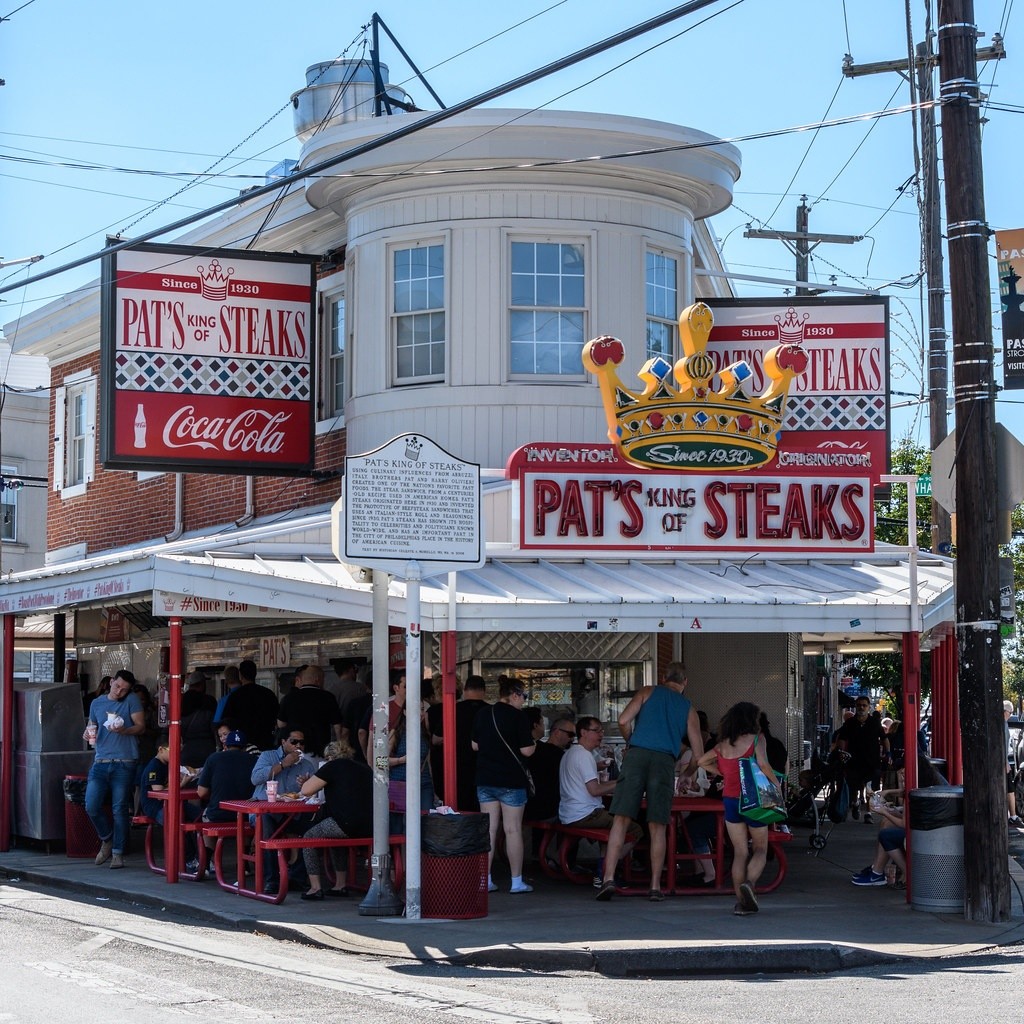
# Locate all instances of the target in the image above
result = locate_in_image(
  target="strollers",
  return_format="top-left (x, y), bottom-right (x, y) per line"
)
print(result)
top-left (785, 743), bottom-right (856, 850)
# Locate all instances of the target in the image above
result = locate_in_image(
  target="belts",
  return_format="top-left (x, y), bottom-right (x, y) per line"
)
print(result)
top-left (96, 758), bottom-right (135, 763)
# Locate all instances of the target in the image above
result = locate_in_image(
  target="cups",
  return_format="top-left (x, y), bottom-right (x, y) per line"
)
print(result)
top-left (600, 771), bottom-right (610, 782)
top-left (266, 781), bottom-right (279, 803)
top-left (86, 725), bottom-right (97, 745)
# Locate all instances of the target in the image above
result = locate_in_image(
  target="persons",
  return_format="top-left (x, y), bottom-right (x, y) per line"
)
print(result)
top-left (595, 661), bottom-right (704, 902)
top-left (697, 701), bottom-right (782, 914)
top-left (82, 669), bottom-right (145, 869)
top-left (426, 671), bottom-right (537, 893)
top-left (180, 660), bottom-right (373, 769)
top-left (82, 676), bottom-right (158, 814)
top-left (1003, 700), bottom-right (1024, 828)
top-left (366, 668), bottom-right (439, 811)
top-left (141, 719), bottom-right (373, 901)
top-left (836, 695), bottom-right (927, 824)
top-left (559, 717), bottom-right (643, 888)
top-left (522, 707), bottom-right (576, 822)
top-left (681, 711), bottom-right (788, 886)
top-left (852, 744), bottom-right (951, 886)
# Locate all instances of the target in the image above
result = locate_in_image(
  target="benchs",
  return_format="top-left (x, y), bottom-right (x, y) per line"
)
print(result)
top-left (131, 816), bottom-right (406, 905)
top-left (721, 830), bottom-right (793, 894)
top-left (521, 820), bottom-right (637, 895)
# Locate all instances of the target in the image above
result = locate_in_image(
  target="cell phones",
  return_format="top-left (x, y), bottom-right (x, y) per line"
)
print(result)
top-left (118, 689), bottom-right (133, 702)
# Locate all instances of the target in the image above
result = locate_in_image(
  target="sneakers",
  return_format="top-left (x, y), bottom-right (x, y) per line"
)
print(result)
top-left (851, 865), bottom-right (887, 886)
top-left (1008, 817), bottom-right (1024, 828)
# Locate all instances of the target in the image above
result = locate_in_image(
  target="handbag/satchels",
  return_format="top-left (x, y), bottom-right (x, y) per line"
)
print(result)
top-left (738, 734), bottom-right (788, 825)
top-left (522, 767), bottom-right (535, 795)
top-left (826, 774), bottom-right (850, 824)
top-left (389, 781), bottom-right (406, 813)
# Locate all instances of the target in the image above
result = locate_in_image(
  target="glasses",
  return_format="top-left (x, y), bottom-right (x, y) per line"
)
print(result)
top-left (519, 690), bottom-right (527, 700)
top-left (290, 739), bottom-right (305, 746)
top-left (857, 703), bottom-right (868, 708)
top-left (586, 728), bottom-right (605, 735)
top-left (554, 728), bottom-right (576, 739)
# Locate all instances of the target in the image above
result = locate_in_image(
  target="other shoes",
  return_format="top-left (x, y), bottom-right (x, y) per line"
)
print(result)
top-left (890, 880), bottom-right (907, 889)
top-left (648, 890), bottom-right (664, 901)
top-left (94, 840), bottom-right (112, 865)
top-left (852, 803), bottom-right (860, 820)
top-left (864, 814), bottom-right (873, 824)
top-left (596, 880), bottom-right (615, 901)
top-left (263, 881), bottom-right (279, 894)
top-left (488, 882), bottom-right (534, 893)
top-left (185, 861), bottom-right (215, 877)
top-left (301, 889), bottom-right (323, 900)
top-left (109, 854), bottom-right (123, 868)
top-left (735, 880), bottom-right (759, 914)
top-left (324, 887), bottom-right (348, 897)
top-left (684, 871), bottom-right (715, 887)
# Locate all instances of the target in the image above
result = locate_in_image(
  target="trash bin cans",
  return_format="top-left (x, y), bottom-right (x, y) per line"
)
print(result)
top-left (910, 785), bottom-right (965, 913)
top-left (403, 810), bottom-right (490, 919)
top-left (65, 775), bottom-right (113, 858)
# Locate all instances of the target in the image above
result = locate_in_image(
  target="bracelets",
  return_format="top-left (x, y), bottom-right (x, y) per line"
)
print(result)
top-left (279, 762), bottom-right (284, 770)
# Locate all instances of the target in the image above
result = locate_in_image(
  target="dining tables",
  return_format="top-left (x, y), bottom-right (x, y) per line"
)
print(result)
top-left (219, 799), bottom-right (396, 900)
top-left (147, 788), bottom-right (211, 881)
top-left (602, 795), bottom-right (726, 893)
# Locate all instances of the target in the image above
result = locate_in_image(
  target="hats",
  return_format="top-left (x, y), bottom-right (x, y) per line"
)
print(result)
top-left (186, 670), bottom-right (212, 685)
top-left (226, 732), bottom-right (244, 746)
